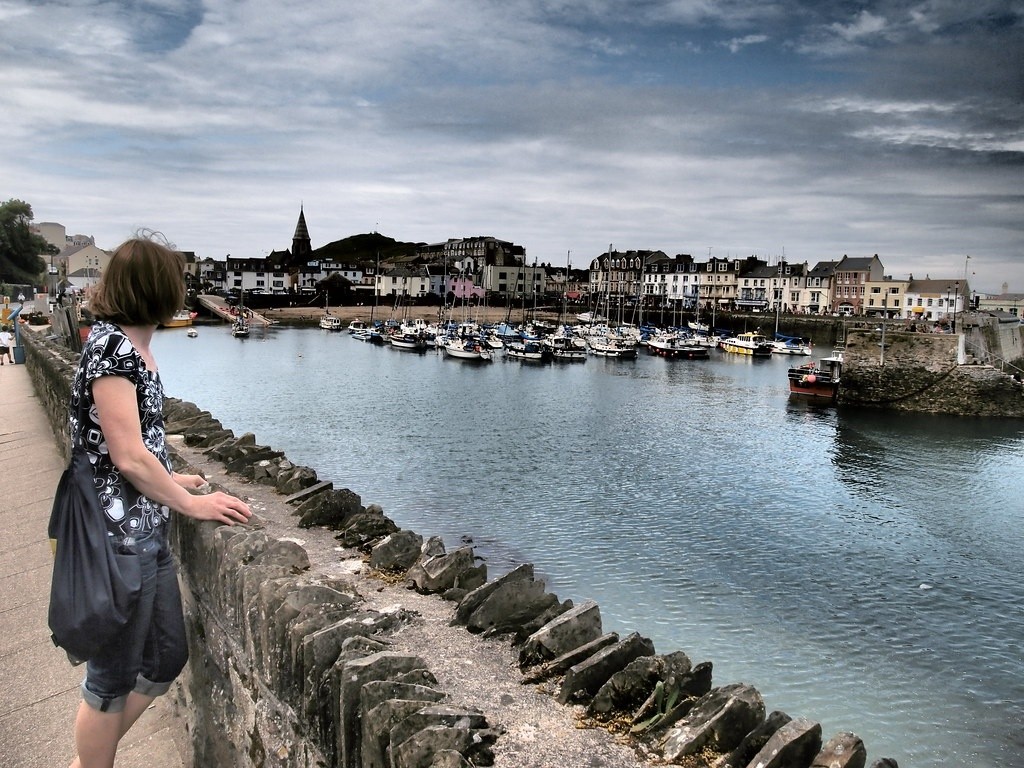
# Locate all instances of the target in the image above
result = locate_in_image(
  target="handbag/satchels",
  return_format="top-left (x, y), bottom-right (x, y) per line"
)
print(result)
top-left (48, 446), bottom-right (142, 666)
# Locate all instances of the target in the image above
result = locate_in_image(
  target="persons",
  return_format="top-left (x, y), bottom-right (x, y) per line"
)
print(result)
top-left (46, 225), bottom-right (254, 768)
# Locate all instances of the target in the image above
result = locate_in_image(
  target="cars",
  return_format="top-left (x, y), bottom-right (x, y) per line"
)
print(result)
top-left (58, 287), bottom-right (75, 302)
top-left (70, 285), bottom-right (84, 300)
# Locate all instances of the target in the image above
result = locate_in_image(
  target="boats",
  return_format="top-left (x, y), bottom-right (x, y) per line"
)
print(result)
top-left (160, 241), bottom-right (846, 397)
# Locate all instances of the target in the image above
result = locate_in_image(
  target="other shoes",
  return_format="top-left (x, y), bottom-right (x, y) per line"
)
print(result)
top-left (9, 360), bottom-right (14, 363)
top-left (0, 363), bottom-right (3, 365)
